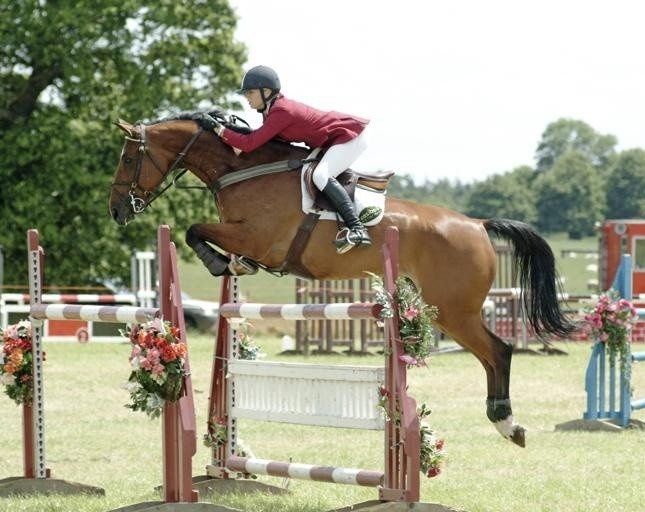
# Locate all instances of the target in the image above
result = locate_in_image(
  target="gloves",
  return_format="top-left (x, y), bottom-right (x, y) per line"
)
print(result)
top-left (192, 112), bottom-right (218, 131)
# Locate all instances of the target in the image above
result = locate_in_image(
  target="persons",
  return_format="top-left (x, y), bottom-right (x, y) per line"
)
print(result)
top-left (192, 64), bottom-right (371, 245)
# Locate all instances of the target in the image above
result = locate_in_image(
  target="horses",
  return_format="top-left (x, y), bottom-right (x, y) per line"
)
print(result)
top-left (108, 108), bottom-right (591, 449)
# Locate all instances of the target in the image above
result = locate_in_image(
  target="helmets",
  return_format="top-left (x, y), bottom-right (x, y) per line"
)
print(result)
top-left (236, 65), bottom-right (281, 94)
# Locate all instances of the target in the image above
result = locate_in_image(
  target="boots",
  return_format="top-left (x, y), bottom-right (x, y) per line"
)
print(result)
top-left (321, 177), bottom-right (372, 247)
